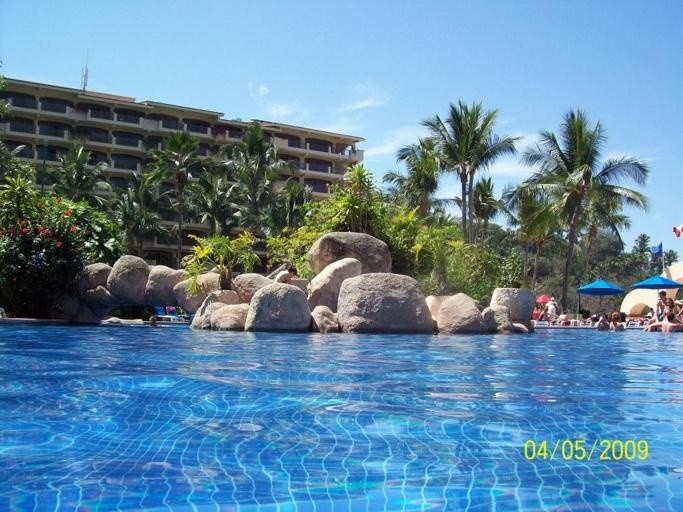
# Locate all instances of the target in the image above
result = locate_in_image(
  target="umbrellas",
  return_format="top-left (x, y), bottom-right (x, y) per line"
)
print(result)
top-left (630, 273), bottom-right (682, 296)
top-left (576, 276), bottom-right (624, 306)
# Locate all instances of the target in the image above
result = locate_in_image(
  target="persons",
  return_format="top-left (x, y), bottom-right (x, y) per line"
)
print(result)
top-left (661, 311), bottom-right (682, 333)
top-left (644, 291), bottom-right (667, 331)
top-left (531, 294), bottom-right (568, 327)
top-left (647, 297), bottom-right (674, 333)
top-left (585, 307), bottom-right (655, 333)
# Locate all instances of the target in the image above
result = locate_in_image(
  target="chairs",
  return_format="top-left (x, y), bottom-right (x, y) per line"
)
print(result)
top-left (154, 306), bottom-right (190, 323)
